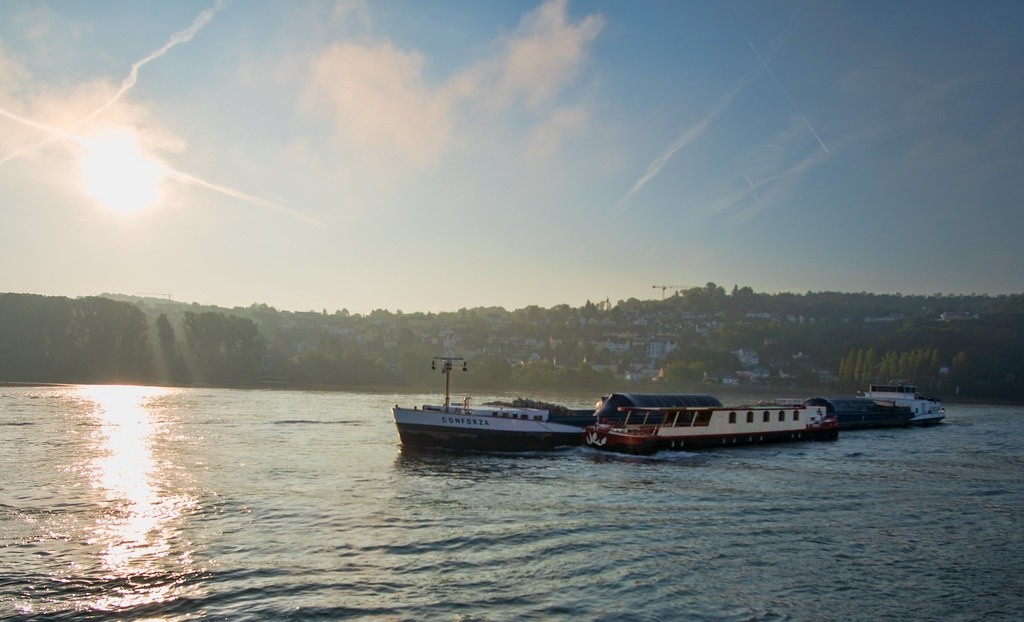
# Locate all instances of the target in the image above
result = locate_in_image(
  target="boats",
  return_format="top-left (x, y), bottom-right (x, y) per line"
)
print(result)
top-left (857, 380), bottom-right (946, 427)
top-left (800, 396), bottom-right (916, 429)
top-left (582, 392), bottom-right (840, 456)
top-left (393, 357), bottom-right (587, 452)
